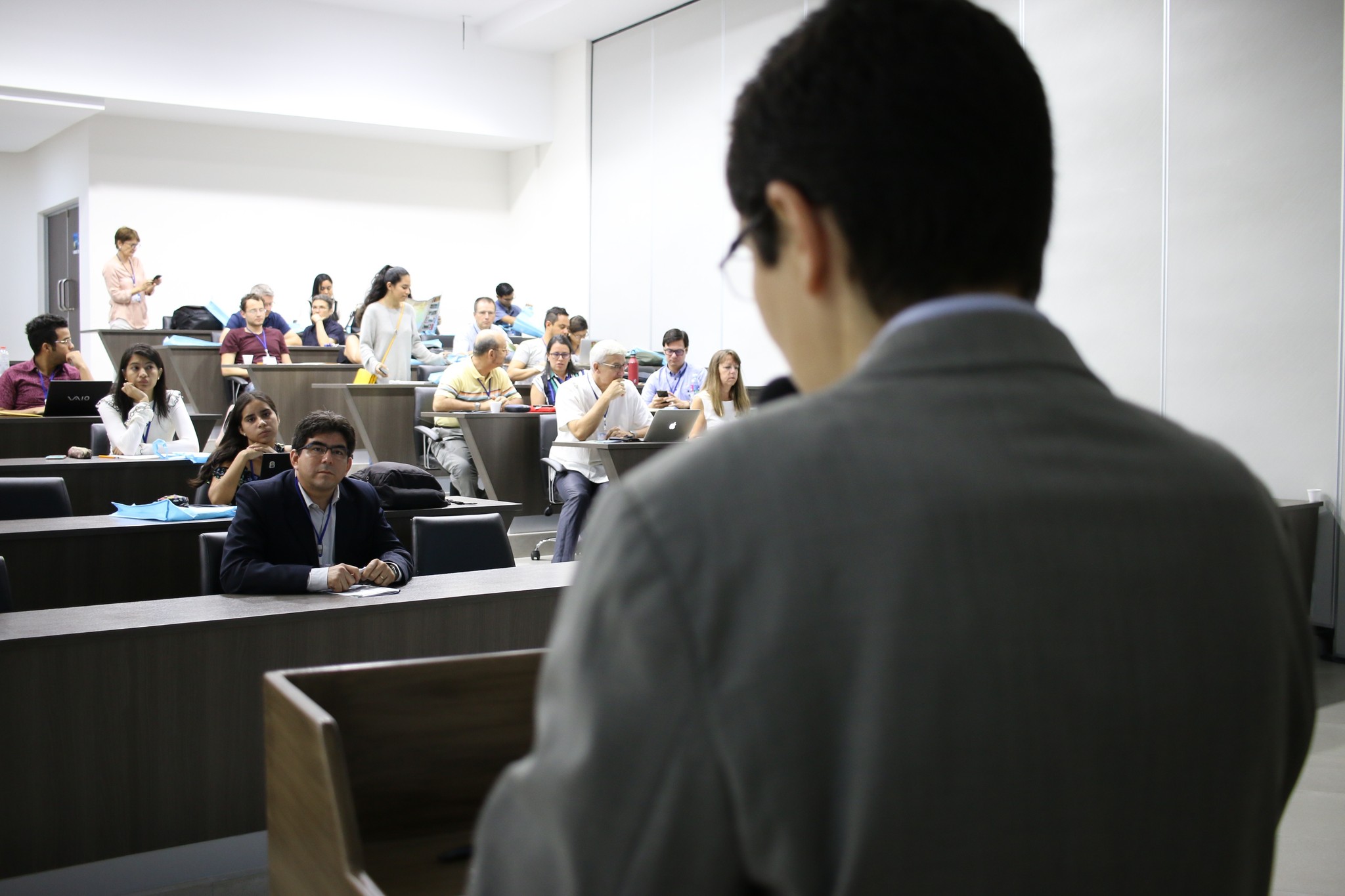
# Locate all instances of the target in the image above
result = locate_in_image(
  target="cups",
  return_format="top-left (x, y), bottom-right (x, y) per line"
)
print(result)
top-left (242, 355), bottom-right (254, 365)
top-left (490, 402), bottom-right (502, 413)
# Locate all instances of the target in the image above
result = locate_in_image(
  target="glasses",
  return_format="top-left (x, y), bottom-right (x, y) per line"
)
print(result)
top-left (548, 352), bottom-right (570, 359)
top-left (296, 442), bottom-right (350, 460)
top-left (572, 331), bottom-right (589, 339)
top-left (46, 338), bottom-right (71, 346)
top-left (244, 308), bottom-right (267, 315)
top-left (719, 206), bottom-right (780, 301)
top-left (500, 294), bottom-right (515, 301)
top-left (491, 348), bottom-right (508, 354)
top-left (311, 306), bottom-right (328, 311)
top-left (123, 242), bottom-right (140, 249)
top-left (601, 363), bottom-right (629, 370)
top-left (663, 347), bottom-right (687, 356)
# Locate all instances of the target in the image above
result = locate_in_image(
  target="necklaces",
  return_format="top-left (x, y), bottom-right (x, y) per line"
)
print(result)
top-left (383, 297), bottom-right (403, 335)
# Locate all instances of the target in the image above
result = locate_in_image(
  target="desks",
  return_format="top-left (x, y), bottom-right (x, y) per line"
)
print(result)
top-left (0, 328), bottom-right (686, 879)
top-left (1276, 499), bottom-right (1324, 606)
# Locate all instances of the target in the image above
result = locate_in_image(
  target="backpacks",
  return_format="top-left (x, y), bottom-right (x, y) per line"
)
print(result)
top-left (348, 462), bottom-right (451, 509)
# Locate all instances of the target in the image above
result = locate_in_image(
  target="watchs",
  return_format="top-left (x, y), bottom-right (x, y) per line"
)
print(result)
top-left (628, 430), bottom-right (638, 438)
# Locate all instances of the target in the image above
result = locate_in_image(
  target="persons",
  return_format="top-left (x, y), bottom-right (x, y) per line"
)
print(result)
top-left (452, 297), bottom-right (519, 365)
top-left (754, 373), bottom-right (799, 411)
top-left (219, 265), bottom-right (452, 392)
top-left (640, 329), bottom-right (708, 409)
top-left (219, 410), bottom-right (415, 596)
top-left (530, 334), bottom-right (589, 408)
top-left (462, 0), bottom-right (1318, 896)
top-left (95, 343), bottom-right (200, 456)
top-left (507, 306), bottom-right (582, 385)
top-left (687, 349), bottom-right (751, 440)
top-left (548, 339), bottom-right (656, 563)
top-left (0, 313), bottom-right (95, 415)
top-left (102, 227), bottom-right (162, 330)
top-left (493, 283), bottom-right (534, 336)
top-left (428, 329), bottom-right (525, 499)
top-left (187, 392), bottom-right (293, 507)
top-left (565, 315), bottom-right (589, 369)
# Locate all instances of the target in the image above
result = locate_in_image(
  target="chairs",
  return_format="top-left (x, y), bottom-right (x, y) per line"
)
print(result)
top-left (0, 477), bottom-right (73, 520)
top-left (198, 532), bottom-right (228, 596)
top-left (194, 465), bottom-right (212, 505)
top-left (420, 335), bottom-right (454, 349)
top-left (91, 423), bottom-right (111, 456)
top-left (411, 512), bottom-right (516, 577)
top-left (417, 365), bottom-right (449, 381)
top-left (414, 386), bottom-right (459, 496)
top-left (225, 376), bottom-right (248, 405)
top-left (531, 414), bottom-right (568, 560)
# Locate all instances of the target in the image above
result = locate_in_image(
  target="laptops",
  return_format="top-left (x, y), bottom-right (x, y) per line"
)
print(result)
top-left (37, 380), bottom-right (112, 416)
top-left (638, 409), bottom-right (701, 441)
top-left (260, 452), bottom-right (294, 480)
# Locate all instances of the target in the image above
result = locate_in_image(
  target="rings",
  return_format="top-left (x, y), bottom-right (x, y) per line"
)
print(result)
top-left (379, 574), bottom-right (385, 581)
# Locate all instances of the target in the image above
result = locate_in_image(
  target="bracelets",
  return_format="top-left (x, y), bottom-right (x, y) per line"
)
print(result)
top-left (474, 402), bottom-right (480, 411)
top-left (686, 400), bottom-right (691, 409)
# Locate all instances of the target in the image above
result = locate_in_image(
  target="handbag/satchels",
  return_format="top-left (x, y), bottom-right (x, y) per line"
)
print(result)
top-left (353, 367), bottom-right (377, 384)
top-left (171, 305), bottom-right (223, 331)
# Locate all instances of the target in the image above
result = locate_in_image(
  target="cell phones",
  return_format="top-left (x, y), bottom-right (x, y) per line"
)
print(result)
top-left (657, 391), bottom-right (670, 404)
top-left (151, 275), bottom-right (161, 284)
top-left (45, 455), bottom-right (67, 459)
top-left (533, 404), bottom-right (553, 407)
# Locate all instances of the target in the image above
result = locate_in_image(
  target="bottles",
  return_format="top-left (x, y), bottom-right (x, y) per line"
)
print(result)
top-left (690, 374), bottom-right (700, 409)
top-left (628, 354), bottom-right (638, 385)
top-left (0, 346), bottom-right (10, 377)
